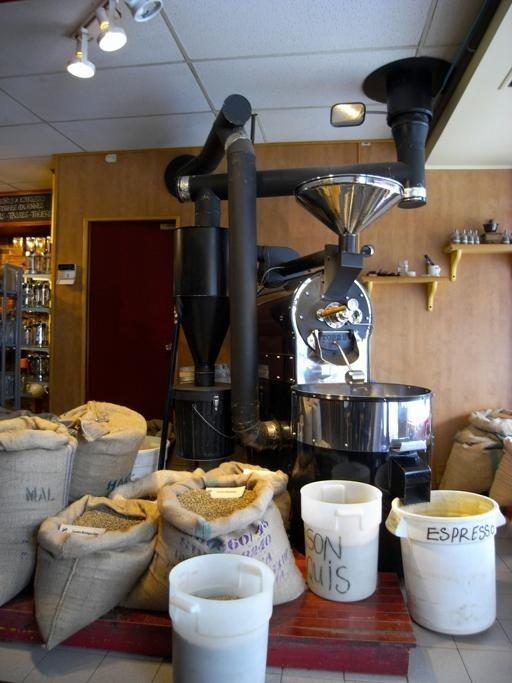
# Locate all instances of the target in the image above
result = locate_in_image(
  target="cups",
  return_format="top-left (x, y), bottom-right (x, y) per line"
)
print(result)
top-left (396, 257), bottom-right (408, 276)
top-left (427, 264), bottom-right (441, 275)
top-left (407, 271), bottom-right (416, 277)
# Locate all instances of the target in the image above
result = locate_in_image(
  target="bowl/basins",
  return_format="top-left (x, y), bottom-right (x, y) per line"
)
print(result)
top-left (482, 223), bottom-right (498, 231)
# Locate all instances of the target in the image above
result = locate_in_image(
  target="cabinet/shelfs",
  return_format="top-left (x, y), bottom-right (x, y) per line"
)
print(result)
top-left (0, 261), bottom-right (52, 413)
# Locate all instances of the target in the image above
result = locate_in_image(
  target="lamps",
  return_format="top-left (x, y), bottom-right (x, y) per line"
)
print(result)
top-left (64, 0), bottom-right (166, 80)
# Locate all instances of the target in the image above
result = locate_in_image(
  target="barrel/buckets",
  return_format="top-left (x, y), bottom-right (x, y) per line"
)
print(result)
top-left (300, 480), bottom-right (383, 603)
top-left (131, 436), bottom-right (170, 483)
top-left (173, 382), bottom-right (236, 462)
top-left (386, 490), bottom-right (506, 637)
top-left (169, 554), bottom-right (274, 683)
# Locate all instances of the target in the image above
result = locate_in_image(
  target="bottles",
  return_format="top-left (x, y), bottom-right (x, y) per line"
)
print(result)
top-left (501, 228), bottom-right (510, 244)
top-left (448, 227), bottom-right (479, 243)
top-left (11, 234), bottom-right (53, 395)
